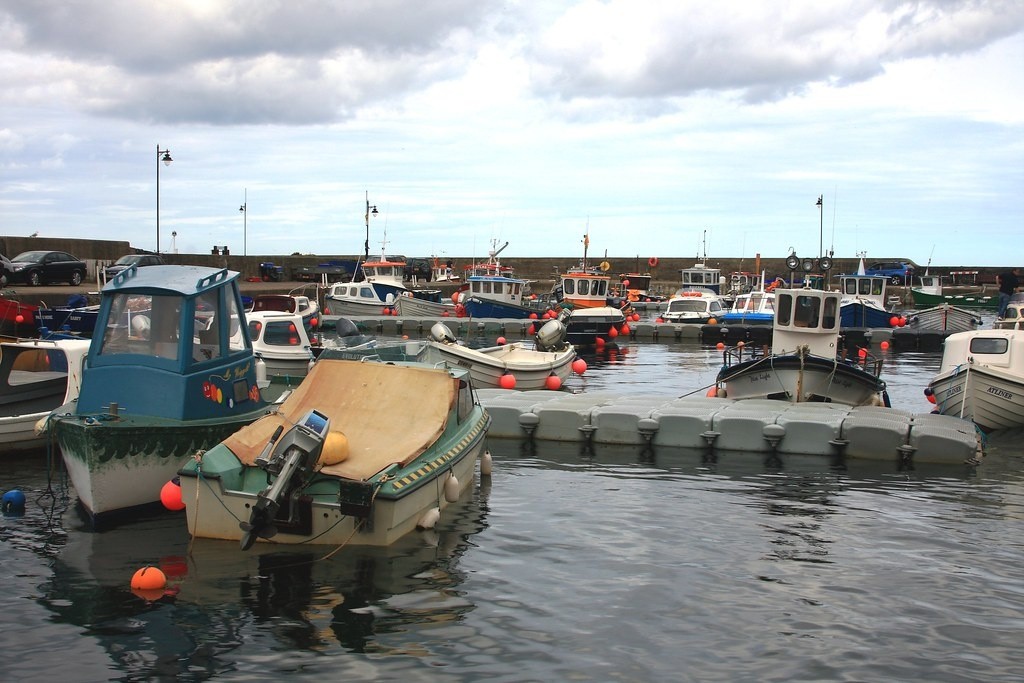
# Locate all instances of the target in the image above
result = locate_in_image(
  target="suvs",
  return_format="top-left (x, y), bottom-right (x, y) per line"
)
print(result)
top-left (851, 261), bottom-right (915, 286)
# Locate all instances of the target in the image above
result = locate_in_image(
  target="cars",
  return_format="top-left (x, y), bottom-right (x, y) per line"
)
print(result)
top-left (99, 255), bottom-right (166, 285)
top-left (10, 251), bottom-right (87, 287)
top-left (403, 257), bottom-right (432, 283)
top-left (361, 255), bottom-right (404, 276)
top-left (0, 255), bottom-right (15, 290)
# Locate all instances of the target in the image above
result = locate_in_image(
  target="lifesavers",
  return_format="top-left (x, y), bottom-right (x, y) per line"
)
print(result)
top-left (766, 280), bottom-right (780, 292)
top-left (882, 391), bottom-right (891, 408)
top-left (681, 291), bottom-right (702, 297)
top-left (648, 256), bottom-right (659, 267)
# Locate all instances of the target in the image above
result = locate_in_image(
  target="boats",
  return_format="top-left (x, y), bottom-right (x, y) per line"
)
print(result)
top-left (661, 229), bottom-right (776, 325)
top-left (0, 338), bottom-right (91, 456)
top-left (715, 252), bottom-right (886, 407)
top-left (176, 335), bottom-right (492, 548)
top-left (416, 319), bottom-right (577, 391)
top-left (906, 302), bottom-right (983, 332)
top-left (194, 283), bottom-right (320, 345)
top-left (0, 293), bottom-right (152, 340)
top-left (430, 220), bottom-right (669, 346)
top-left (47, 262), bottom-right (296, 529)
top-left (929, 329), bottom-right (1024, 433)
top-left (176, 310), bottom-right (466, 386)
top-left (994, 291), bottom-right (1024, 331)
top-left (325, 218), bottom-right (412, 316)
top-left (394, 295), bottom-right (457, 317)
top-left (833, 250), bottom-right (901, 328)
top-left (910, 244), bottom-right (1000, 309)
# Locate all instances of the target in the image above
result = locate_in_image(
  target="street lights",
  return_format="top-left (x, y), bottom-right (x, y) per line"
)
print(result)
top-left (239, 203), bottom-right (246, 255)
top-left (366, 200), bottom-right (379, 262)
top-left (814, 194), bottom-right (823, 258)
top-left (157, 144), bottom-right (173, 255)
